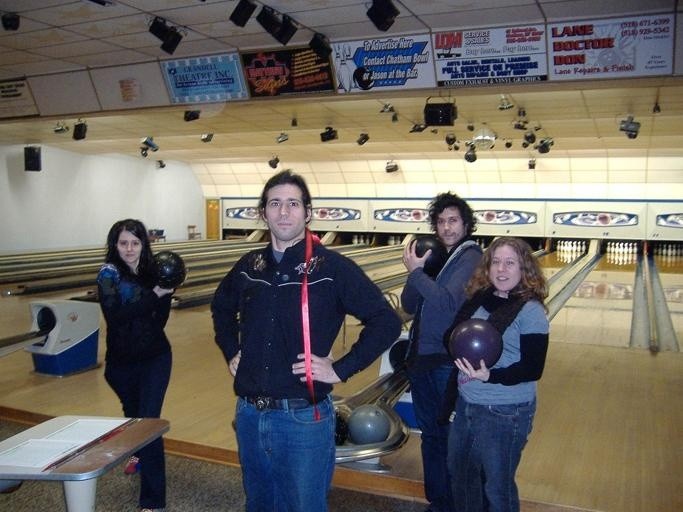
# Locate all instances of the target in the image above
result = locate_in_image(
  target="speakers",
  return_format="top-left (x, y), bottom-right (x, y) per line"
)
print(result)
top-left (424, 102), bottom-right (457, 127)
top-left (25, 146), bottom-right (41, 171)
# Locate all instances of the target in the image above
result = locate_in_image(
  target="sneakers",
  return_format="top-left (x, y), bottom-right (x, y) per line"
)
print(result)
top-left (124, 456), bottom-right (141, 474)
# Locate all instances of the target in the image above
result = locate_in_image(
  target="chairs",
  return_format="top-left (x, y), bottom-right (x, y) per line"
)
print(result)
top-left (154, 229), bottom-right (167, 242)
top-left (187, 225), bottom-right (201, 241)
top-left (147, 229), bottom-right (155, 242)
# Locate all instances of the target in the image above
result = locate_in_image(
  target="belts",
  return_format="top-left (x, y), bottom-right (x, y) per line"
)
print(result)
top-left (242, 395), bottom-right (310, 410)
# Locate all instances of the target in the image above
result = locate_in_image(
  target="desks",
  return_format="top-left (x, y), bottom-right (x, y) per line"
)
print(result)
top-left (0, 415), bottom-right (171, 511)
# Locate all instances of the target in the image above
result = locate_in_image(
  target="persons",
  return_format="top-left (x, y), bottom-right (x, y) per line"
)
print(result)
top-left (204, 167), bottom-right (404, 511)
top-left (444, 235), bottom-right (550, 512)
top-left (398, 190), bottom-right (484, 511)
top-left (96, 218), bottom-right (189, 512)
